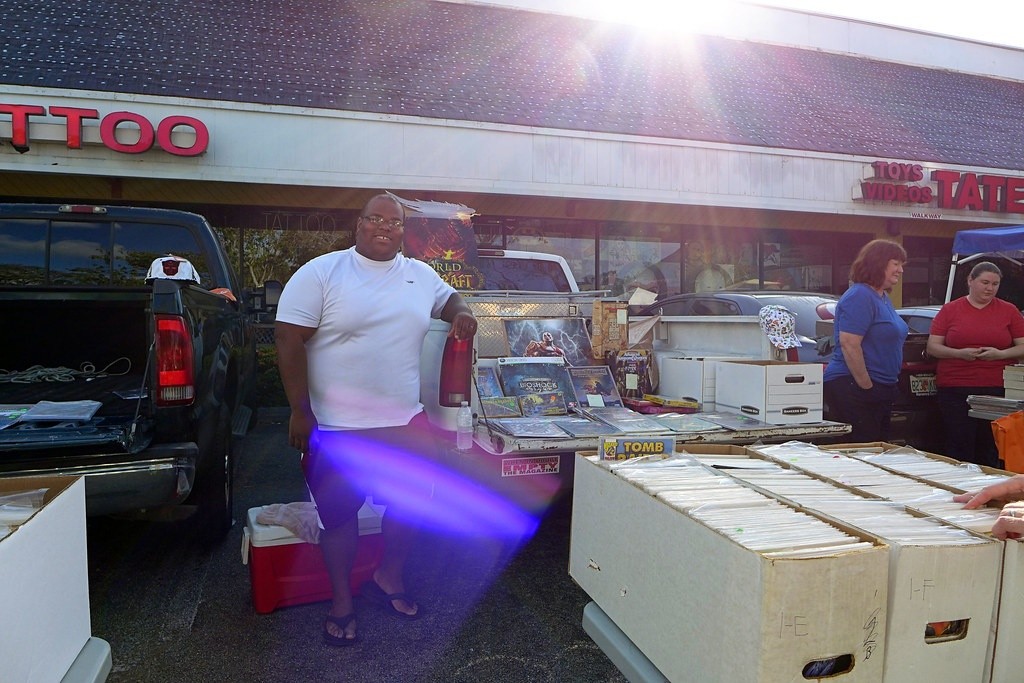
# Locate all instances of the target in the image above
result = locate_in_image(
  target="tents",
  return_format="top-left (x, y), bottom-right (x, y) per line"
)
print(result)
top-left (941, 226), bottom-right (1024, 304)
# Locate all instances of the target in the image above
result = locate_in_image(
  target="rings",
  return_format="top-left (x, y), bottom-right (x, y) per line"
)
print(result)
top-left (470, 326), bottom-right (474, 328)
top-left (1011, 510), bottom-right (1015, 517)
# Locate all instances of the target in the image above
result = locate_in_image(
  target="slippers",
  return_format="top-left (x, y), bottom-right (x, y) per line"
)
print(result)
top-left (359, 579), bottom-right (421, 620)
top-left (324, 598), bottom-right (358, 646)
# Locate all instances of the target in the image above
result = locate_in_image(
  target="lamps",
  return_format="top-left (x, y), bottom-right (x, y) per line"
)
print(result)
top-left (887, 219), bottom-right (901, 238)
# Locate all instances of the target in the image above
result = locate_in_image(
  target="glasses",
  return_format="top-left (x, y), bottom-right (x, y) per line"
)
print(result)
top-left (361, 214), bottom-right (404, 232)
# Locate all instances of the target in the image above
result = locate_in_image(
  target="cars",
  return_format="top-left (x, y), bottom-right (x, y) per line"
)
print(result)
top-left (631, 290), bottom-right (942, 442)
top-left (894, 305), bottom-right (944, 334)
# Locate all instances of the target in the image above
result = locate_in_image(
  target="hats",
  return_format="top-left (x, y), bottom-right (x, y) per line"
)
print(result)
top-left (144, 254), bottom-right (201, 286)
top-left (759, 305), bottom-right (802, 350)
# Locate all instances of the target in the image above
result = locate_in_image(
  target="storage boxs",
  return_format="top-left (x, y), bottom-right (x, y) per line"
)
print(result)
top-left (0, 474), bottom-right (92, 683)
top-left (241, 501), bottom-right (390, 613)
top-left (661, 356), bottom-right (822, 425)
top-left (568, 441), bottom-right (1024, 683)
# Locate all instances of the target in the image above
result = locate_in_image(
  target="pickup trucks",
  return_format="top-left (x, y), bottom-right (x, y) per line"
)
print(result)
top-left (0, 203), bottom-right (250, 534)
top-left (411, 247), bottom-right (851, 514)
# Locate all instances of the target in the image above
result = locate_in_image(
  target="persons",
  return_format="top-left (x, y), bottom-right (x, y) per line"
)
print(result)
top-left (927, 261), bottom-right (1024, 465)
top-left (274, 194), bottom-right (477, 646)
top-left (525, 332), bottom-right (565, 358)
top-left (953, 473), bottom-right (1024, 541)
top-left (823, 239), bottom-right (908, 442)
